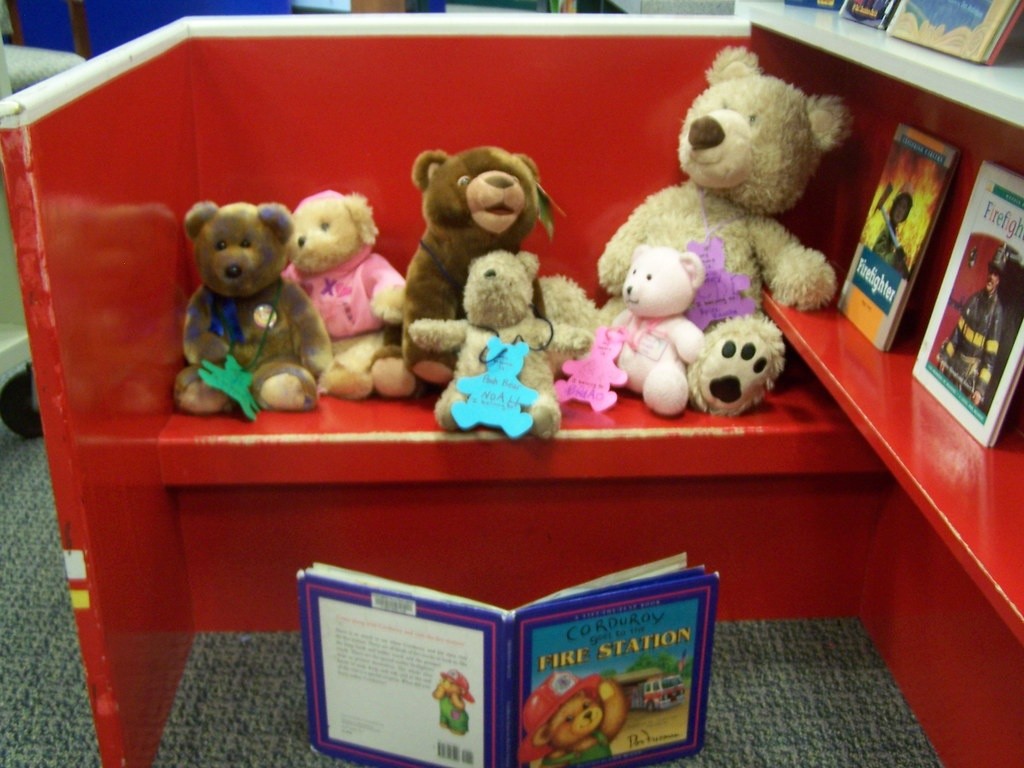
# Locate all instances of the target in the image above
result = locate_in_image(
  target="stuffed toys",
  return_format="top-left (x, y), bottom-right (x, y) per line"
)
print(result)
top-left (167, 44), bottom-right (856, 438)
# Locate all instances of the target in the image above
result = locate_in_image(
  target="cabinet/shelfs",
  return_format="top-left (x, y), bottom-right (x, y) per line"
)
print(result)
top-left (0, 1), bottom-right (1023, 766)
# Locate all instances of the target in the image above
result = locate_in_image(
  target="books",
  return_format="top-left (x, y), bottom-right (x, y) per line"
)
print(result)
top-left (884, 0), bottom-right (1024, 66)
top-left (838, 0), bottom-right (898, 31)
top-left (784, 0), bottom-right (848, 12)
top-left (296, 551), bottom-right (721, 768)
top-left (909, 159), bottom-right (1024, 449)
top-left (836, 121), bottom-right (962, 353)
